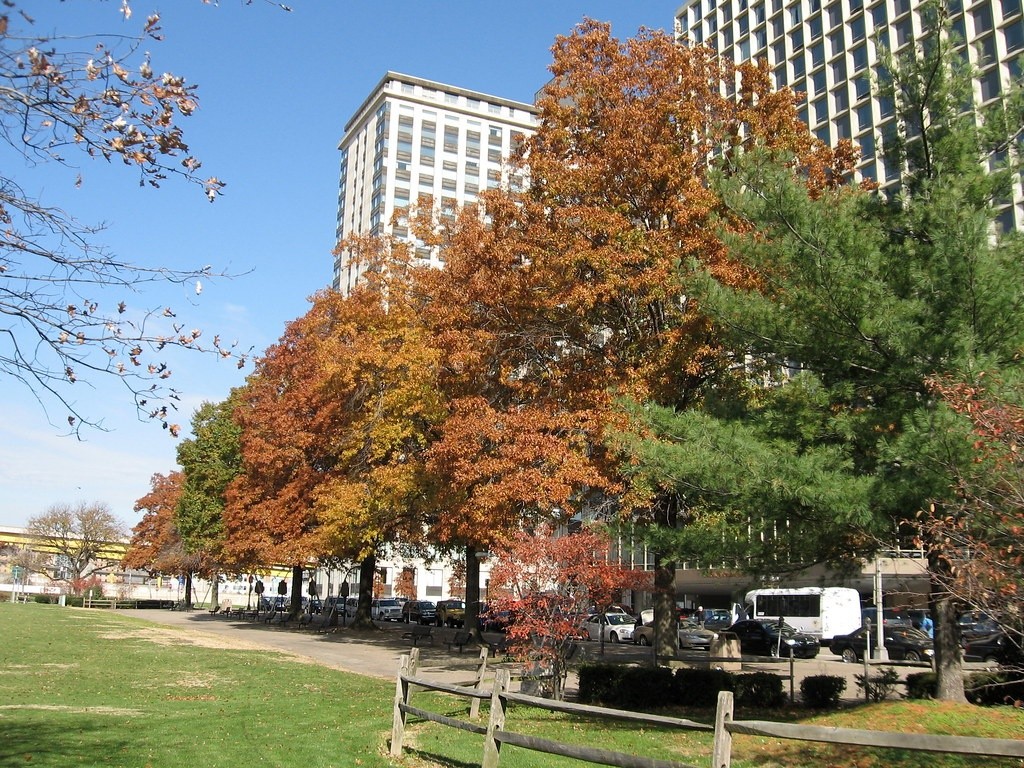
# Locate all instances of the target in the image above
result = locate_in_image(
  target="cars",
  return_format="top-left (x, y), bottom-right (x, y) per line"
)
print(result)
top-left (688, 609), bottom-right (731, 632)
top-left (966, 631), bottom-right (1024, 663)
top-left (576, 613), bottom-right (637, 643)
top-left (479, 601), bottom-right (516, 632)
top-left (588, 603), bottom-right (634, 615)
top-left (721, 619), bottom-right (821, 657)
top-left (257, 596), bottom-right (287, 611)
top-left (307, 599), bottom-right (325, 615)
top-left (862, 606), bottom-right (912, 626)
top-left (909, 609), bottom-right (932, 628)
top-left (345, 598), bottom-right (359, 617)
top-left (961, 612), bottom-right (1003, 649)
top-left (633, 609), bottom-right (719, 649)
top-left (830, 624), bottom-right (966, 663)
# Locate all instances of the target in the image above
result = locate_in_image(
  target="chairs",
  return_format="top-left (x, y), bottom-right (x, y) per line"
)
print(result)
top-left (298, 617), bottom-right (313, 628)
top-left (209, 606), bottom-right (220, 616)
top-left (234, 608), bottom-right (242, 619)
top-left (279, 613), bottom-right (292, 626)
top-left (264, 612), bottom-right (276, 624)
top-left (220, 607), bottom-right (230, 617)
top-left (248, 610), bottom-right (259, 622)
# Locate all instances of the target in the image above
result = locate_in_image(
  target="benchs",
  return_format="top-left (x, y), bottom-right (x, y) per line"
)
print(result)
top-left (443, 632), bottom-right (472, 653)
top-left (402, 625), bottom-right (433, 645)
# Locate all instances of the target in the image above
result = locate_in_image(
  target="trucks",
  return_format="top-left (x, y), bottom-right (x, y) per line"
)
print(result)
top-left (745, 587), bottom-right (862, 640)
top-left (284, 597), bottom-right (308, 613)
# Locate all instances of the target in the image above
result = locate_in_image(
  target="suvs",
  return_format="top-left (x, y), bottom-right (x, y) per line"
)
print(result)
top-left (435, 600), bottom-right (465, 628)
top-left (402, 600), bottom-right (436, 625)
top-left (368, 598), bottom-right (403, 621)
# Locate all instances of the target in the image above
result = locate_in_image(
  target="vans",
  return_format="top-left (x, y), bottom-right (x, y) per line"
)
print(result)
top-left (324, 595), bottom-right (348, 616)
